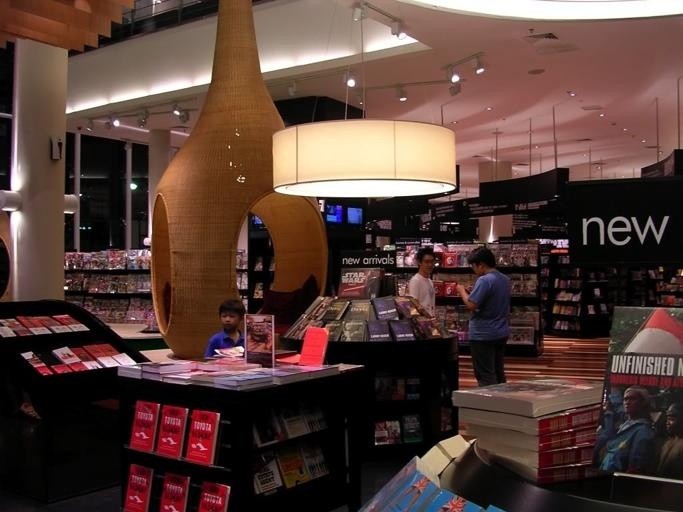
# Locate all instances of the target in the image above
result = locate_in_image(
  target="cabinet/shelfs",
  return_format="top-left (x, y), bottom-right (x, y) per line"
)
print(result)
top-left (118, 368), bottom-right (361, 512)
top-left (280, 331), bottom-right (459, 470)
top-left (394, 241), bottom-right (545, 357)
top-left (541, 248), bottom-right (683, 339)
top-left (0, 299), bottom-right (150, 504)
top-left (64, 251), bottom-right (248, 324)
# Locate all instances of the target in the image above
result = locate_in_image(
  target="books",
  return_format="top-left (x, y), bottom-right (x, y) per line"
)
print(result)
top-left (124, 464), bottom-right (154, 511)
top-left (156, 405), bottom-right (189, 460)
top-left (159, 472), bottom-right (190, 512)
top-left (18, 342), bottom-right (137, 376)
top-left (249, 397), bottom-right (330, 448)
top-left (130, 401), bottom-right (160, 453)
top-left (185, 409), bottom-right (220, 465)
top-left (252, 436), bottom-right (332, 496)
top-left (197, 481), bottom-right (231, 512)
top-left (452, 377), bottom-right (603, 487)
top-left (116, 328), bottom-right (339, 388)
top-left (282, 295), bottom-right (446, 344)
top-left (375, 373), bottom-right (428, 400)
top-left (0, 312), bottom-right (91, 337)
top-left (367, 413), bottom-right (423, 446)
top-left (356, 434), bottom-right (504, 512)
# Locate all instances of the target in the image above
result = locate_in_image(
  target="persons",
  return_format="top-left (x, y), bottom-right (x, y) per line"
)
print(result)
top-left (408, 247), bottom-right (437, 319)
top-left (596, 384), bottom-right (683, 484)
top-left (456, 245), bottom-right (511, 390)
top-left (205, 298), bottom-right (244, 361)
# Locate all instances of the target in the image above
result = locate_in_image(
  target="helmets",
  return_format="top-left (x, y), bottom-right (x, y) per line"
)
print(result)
top-left (665, 402), bottom-right (683, 416)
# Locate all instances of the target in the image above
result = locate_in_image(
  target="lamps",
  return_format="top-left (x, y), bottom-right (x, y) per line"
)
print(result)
top-left (272, 0), bottom-right (456, 198)
top-left (355, 83), bottom-right (461, 105)
top-left (449, 55), bottom-right (485, 83)
top-left (353, 7), bottom-right (402, 37)
top-left (86, 104), bottom-right (200, 131)
top-left (288, 69), bottom-right (356, 97)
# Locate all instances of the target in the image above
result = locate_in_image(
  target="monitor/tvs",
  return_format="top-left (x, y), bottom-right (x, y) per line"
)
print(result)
top-left (251, 215), bottom-right (262, 226)
top-left (325, 204), bottom-right (344, 224)
top-left (347, 206), bottom-right (363, 225)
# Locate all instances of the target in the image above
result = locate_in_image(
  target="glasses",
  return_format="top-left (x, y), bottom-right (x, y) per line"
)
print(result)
top-left (470, 264), bottom-right (479, 269)
